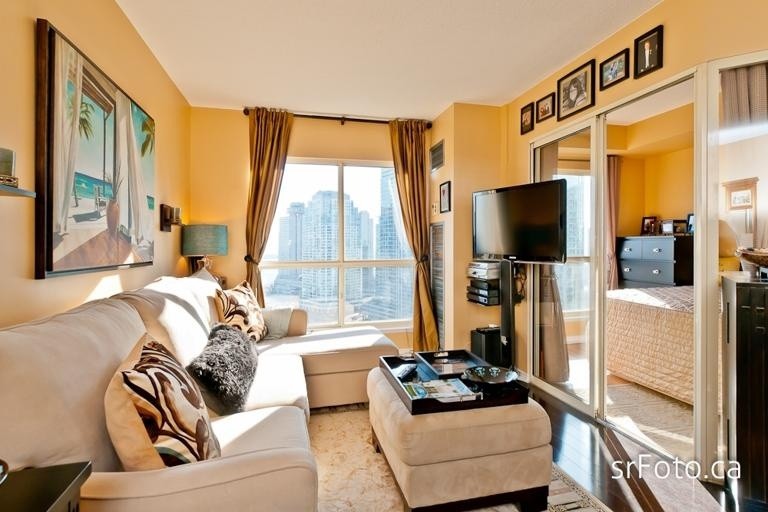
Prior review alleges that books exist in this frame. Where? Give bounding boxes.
[403,378,473,400]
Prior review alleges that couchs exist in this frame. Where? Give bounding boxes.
[0,269,400,512]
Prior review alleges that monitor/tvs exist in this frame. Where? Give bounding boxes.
[472,180,567,265]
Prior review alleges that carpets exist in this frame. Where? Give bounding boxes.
[605,381,695,469]
[309,403,616,512]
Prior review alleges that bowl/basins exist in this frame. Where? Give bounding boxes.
[736,248,768,266]
[462,364,519,386]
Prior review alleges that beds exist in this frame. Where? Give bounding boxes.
[604,285,696,409]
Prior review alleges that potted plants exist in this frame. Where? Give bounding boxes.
[104,161,125,235]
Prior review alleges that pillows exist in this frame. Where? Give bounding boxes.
[214,278,269,343]
[186,323,262,415]
[101,332,222,473]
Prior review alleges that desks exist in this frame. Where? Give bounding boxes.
[0,460,93,512]
[53,228,145,271]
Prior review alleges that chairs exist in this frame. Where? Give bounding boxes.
[93,185,109,218]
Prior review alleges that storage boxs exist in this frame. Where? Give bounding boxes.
[661,219,686,236]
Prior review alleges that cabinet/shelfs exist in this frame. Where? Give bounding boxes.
[721,268,768,511]
[615,232,695,289]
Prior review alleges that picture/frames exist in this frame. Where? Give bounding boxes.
[686,213,695,235]
[437,179,452,213]
[633,22,666,82]
[519,100,536,138]
[640,216,659,238]
[32,16,158,282]
[535,91,556,124]
[598,46,631,93]
[555,56,597,123]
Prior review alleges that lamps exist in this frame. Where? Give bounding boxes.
[181,223,230,279]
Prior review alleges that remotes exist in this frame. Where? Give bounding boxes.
[397,364,417,380]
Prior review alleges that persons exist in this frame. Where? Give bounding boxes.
[540,103,550,117]
[609,61,617,79]
[640,40,657,71]
[568,78,585,108]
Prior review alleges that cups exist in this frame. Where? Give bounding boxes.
[742,258,760,281]
[650,224,659,233]
